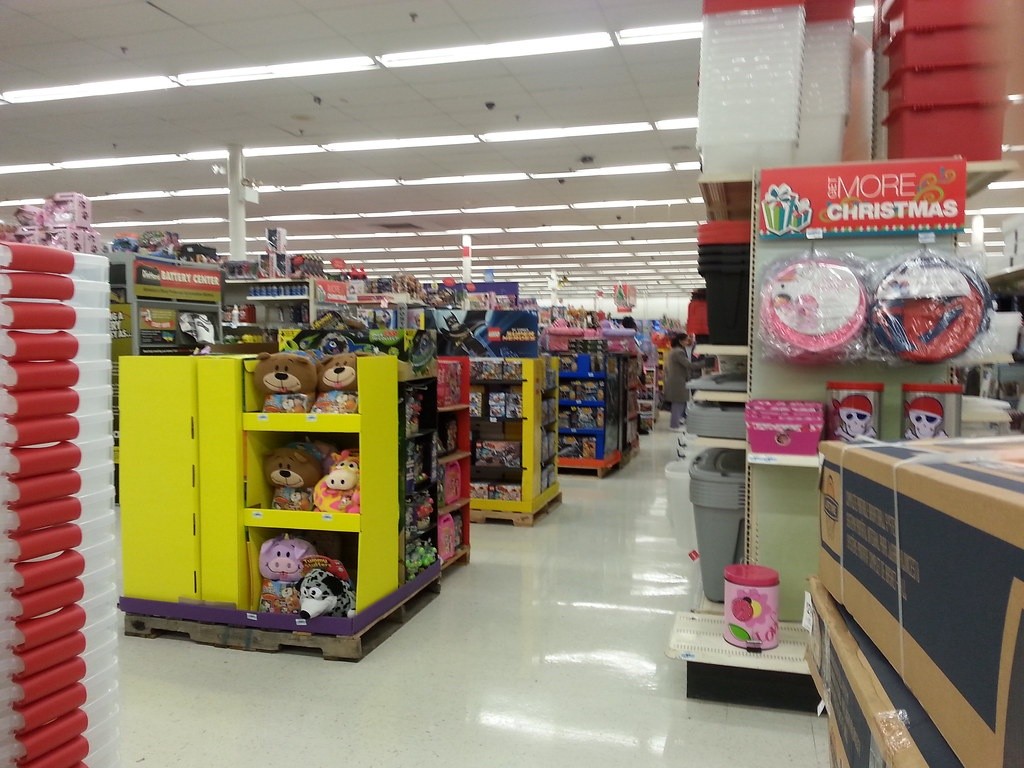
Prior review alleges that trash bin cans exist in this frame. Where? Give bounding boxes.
[688,447,746,604]
[686,219,751,345]
[721,564,779,653]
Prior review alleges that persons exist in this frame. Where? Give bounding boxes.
[663,333,715,432]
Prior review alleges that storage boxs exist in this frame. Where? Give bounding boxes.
[548,350,578,371]
[482,441,521,468]
[576,380,606,402]
[489,406,505,418]
[43,192,92,229]
[84,227,102,253]
[482,361,502,379]
[260,254,290,277]
[686,0,1024,768]
[559,436,597,460]
[469,391,484,418]
[570,406,605,429]
[45,229,85,253]
[488,392,506,405]
[470,483,489,499]
[506,394,524,419]
[489,483,521,500]
[470,361,483,380]
[503,361,522,379]
[265,227,287,254]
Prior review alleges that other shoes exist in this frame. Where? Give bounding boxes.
[669,428,685,432]
[680,417,685,425]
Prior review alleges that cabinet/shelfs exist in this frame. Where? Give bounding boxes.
[7,190,673,661]
[686,163,1017,713]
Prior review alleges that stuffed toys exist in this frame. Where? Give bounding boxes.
[252,349,369,413]
[257,533,356,619]
[262,438,360,514]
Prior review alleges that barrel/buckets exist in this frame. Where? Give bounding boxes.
[689,447,744,600]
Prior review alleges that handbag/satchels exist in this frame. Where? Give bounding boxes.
[684,388,695,415]
